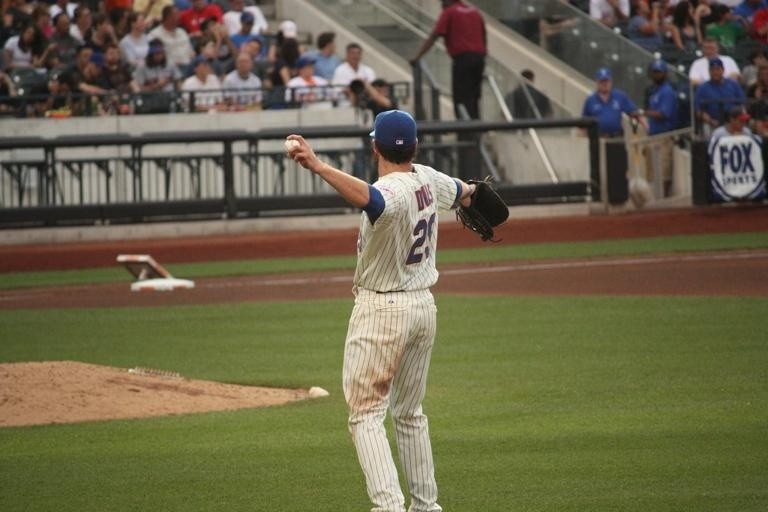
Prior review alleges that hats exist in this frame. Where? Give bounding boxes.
[296,57,316,69]
[596,69,611,82]
[709,58,722,66]
[278,20,297,39]
[370,110,417,145]
[649,60,667,73]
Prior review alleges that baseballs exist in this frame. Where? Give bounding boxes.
[283,139,300,154]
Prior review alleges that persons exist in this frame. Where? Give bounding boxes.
[347,75,399,214]
[538,0,766,209]
[407,1,491,124]
[0,0,399,121]
[284,108,512,512]
[497,68,556,121]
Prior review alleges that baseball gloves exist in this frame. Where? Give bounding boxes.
[453,175,510,242]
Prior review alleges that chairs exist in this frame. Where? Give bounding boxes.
[565,0,768,139]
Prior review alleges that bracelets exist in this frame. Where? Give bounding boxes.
[459,183,473,202]
